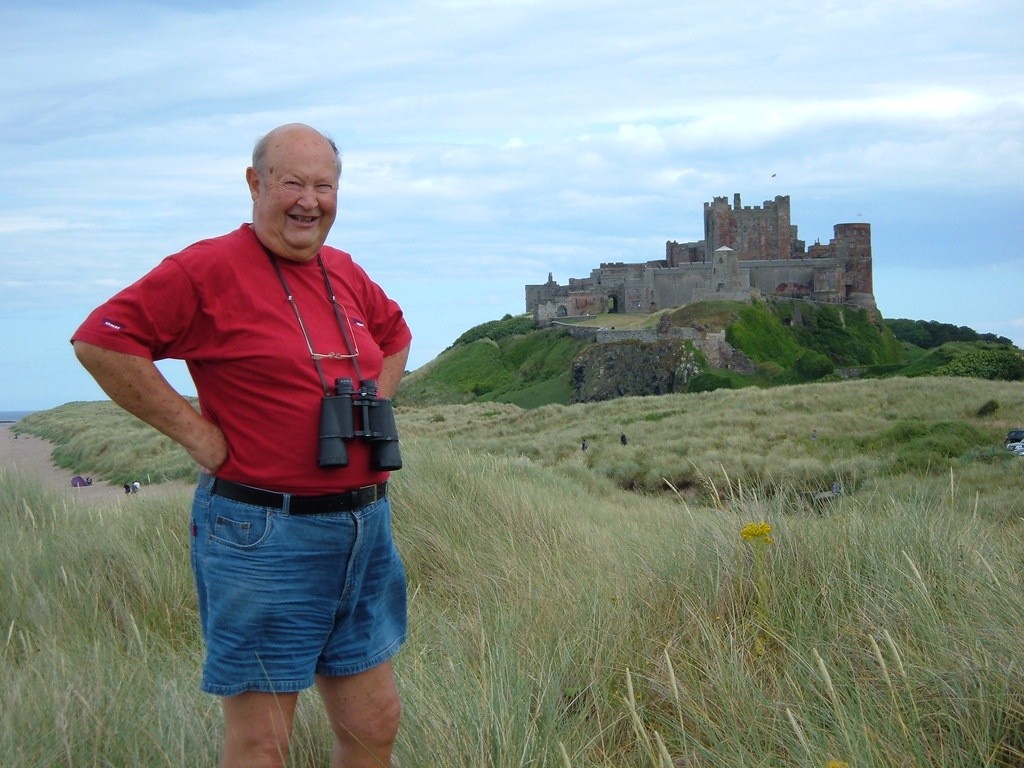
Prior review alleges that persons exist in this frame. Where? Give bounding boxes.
[124,481,141,493]
[71,123,412,768]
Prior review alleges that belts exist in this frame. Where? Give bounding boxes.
[199,471,388,516]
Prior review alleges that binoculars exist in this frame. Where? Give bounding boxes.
[318,377,402,471]
